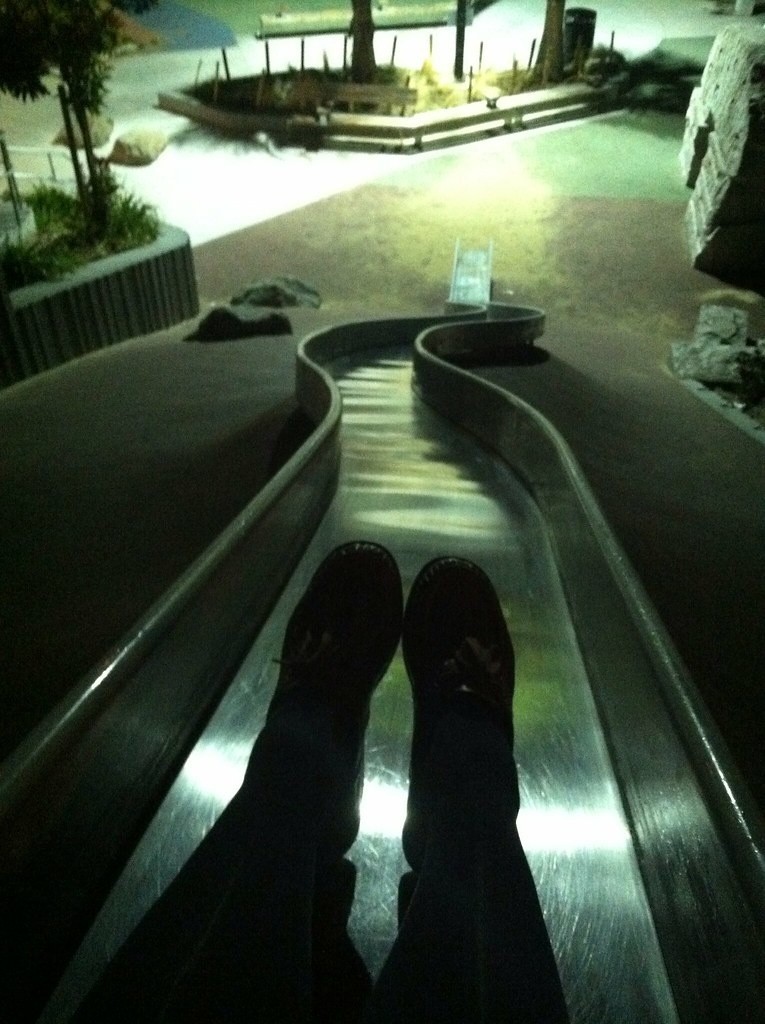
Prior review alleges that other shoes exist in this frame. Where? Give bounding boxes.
[246,540,401,805]
[401,556,520,818]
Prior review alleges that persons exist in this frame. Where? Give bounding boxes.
[68,541,569,1024]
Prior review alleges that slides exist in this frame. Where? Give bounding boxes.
[0,300,764,1024]
